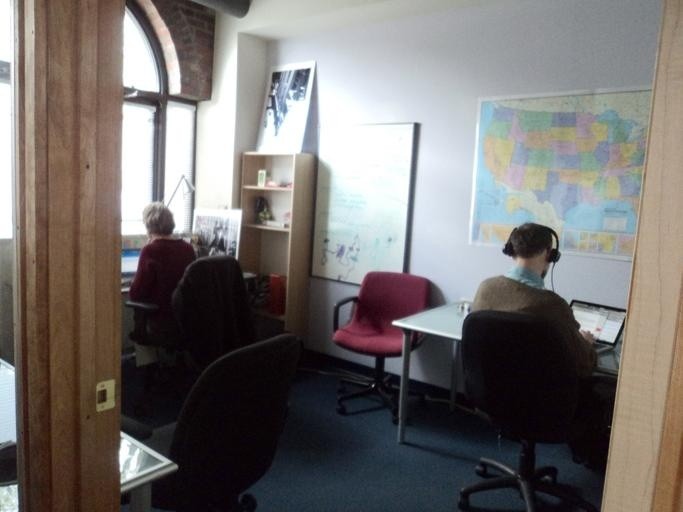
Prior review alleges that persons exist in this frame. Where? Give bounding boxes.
[130,201,197,341]
[467,222,597,379]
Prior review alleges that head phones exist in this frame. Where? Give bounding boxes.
[502,223,561,261]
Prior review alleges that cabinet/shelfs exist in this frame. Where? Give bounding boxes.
[237,151,316,333]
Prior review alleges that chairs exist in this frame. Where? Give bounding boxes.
[121,356,176,434]
[331,270,433,427]
[124,252,242,380]
[123,338,301,510]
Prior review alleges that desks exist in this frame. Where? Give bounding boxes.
[392,301,621,446]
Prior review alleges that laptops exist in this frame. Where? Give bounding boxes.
[121,249,141,286]
[569,300,626,354]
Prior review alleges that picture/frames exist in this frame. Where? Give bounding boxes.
[308,122,419,287]
[468,84,654,263]
[255,60,318,154]
